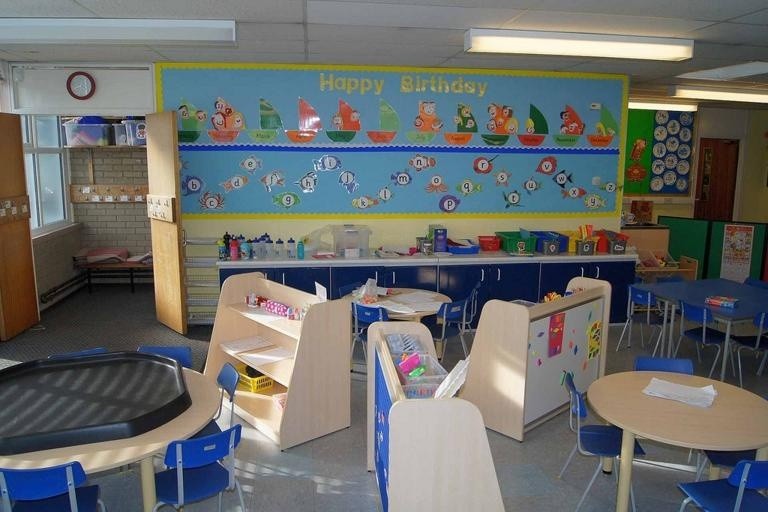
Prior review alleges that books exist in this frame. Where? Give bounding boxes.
[704,296,741,310]
[371,301,415,314]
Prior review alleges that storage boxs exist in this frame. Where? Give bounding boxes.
[121,118,146,147]
[63,121,111,149]
[111,123,128,147]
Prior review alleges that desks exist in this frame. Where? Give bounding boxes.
[1,352,225,510]
[586,364,767,511]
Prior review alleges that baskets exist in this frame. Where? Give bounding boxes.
[393,353,449,398]
[234,363,274,392]
[478,227,630,253]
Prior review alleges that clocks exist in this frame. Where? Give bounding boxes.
[65,70,95,101]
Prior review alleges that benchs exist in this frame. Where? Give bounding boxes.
[75,262,153,293]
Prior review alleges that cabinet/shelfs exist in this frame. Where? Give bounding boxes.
[540,261,637,325]
[438,263,541,306]
[332,267,438,300]
[361,316,505,511]
[218,264,331,302]
[457,273,614,445]
[199,270,357,451]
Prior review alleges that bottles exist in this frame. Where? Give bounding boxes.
[217,230,305,260]
[248,287,257,305]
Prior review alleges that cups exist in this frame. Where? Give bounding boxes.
[423,243,433,256]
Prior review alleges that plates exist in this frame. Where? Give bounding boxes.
[434,250,453,256]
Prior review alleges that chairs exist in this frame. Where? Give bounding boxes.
[615,273,767,390]
[337,274,485,378]
[138,343,194,369]
[145,423,251,511]
[673,459,767,511]
[47,345,109,357]
[155,360,242,471]
[560,368,648,511]
[1,456,108,511]
